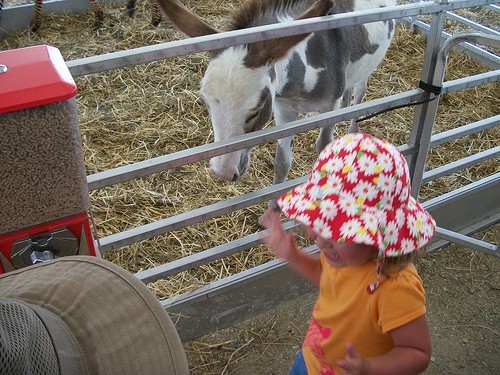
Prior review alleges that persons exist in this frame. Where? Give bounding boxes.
[259,133,437,374]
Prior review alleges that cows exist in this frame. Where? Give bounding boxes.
[159,0,396,228]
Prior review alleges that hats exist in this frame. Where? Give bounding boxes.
[0,255,189,375]
[276,132,436,294]
